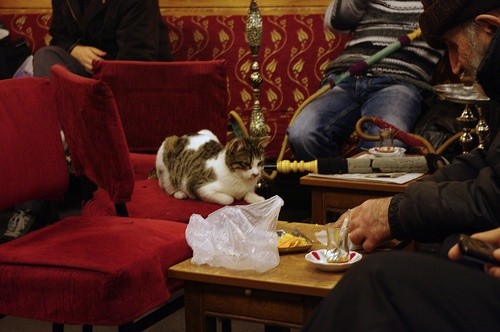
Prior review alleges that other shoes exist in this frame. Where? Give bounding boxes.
[0,200,60,245]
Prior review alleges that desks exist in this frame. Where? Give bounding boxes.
[300,149,428,224]
[167,221,411,332]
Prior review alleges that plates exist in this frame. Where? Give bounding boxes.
[369,146,407,157]
[278,230,314,254]
[305,249,362,272]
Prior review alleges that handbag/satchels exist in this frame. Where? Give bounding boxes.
[185,194,285,273]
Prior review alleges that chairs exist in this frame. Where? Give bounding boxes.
[0,58,251,332]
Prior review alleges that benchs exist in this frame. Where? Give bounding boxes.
[0,13,458,159]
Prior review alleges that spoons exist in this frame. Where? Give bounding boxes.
[327,208,351,262]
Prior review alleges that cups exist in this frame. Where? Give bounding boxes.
[325,223,350,263]
[377,129,394,152]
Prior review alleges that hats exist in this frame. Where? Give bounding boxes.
[419,0,500,50]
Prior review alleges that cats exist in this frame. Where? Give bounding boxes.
[155,128,273,205]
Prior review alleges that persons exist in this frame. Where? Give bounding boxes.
[32,0,172,80]
[286,0,446,161]
[334,0,500,332]
